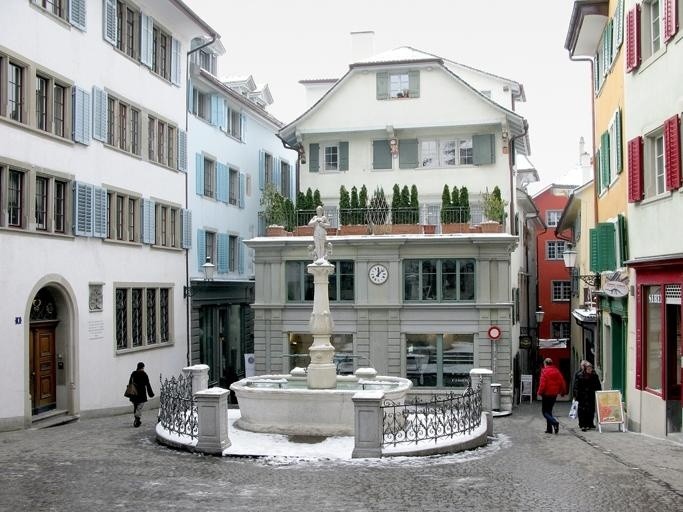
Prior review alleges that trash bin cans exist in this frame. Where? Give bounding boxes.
[490,384,501,411]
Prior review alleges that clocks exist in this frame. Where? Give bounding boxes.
[368,263,389,285]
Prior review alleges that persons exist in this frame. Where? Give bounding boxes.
[308,205,330,264]
[127,362,155,427]
[537,358,567,434]
[573,362,602,430]
[572,359,596,428]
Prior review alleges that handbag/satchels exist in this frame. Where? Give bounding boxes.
[569,401,579,420]
[124,383,139,397]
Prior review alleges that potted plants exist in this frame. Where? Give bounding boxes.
[439,184,471,233]
[480,185,508,233]
[255,182,323,236]
[338,184,422,235]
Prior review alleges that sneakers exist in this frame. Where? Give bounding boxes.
[545,422,558,433]
[581,424,596,430]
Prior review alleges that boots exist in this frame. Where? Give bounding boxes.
[134,417,141,427]
